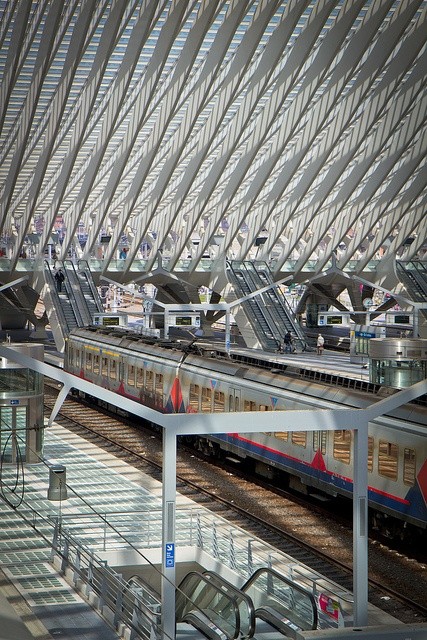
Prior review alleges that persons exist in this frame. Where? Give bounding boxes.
[283,329,295,354]
[316,332,324,354]
[54,269,65,294]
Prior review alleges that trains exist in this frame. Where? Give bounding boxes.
[63,324,426,564]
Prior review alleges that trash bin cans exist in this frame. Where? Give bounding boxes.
[48,464,66,501]
